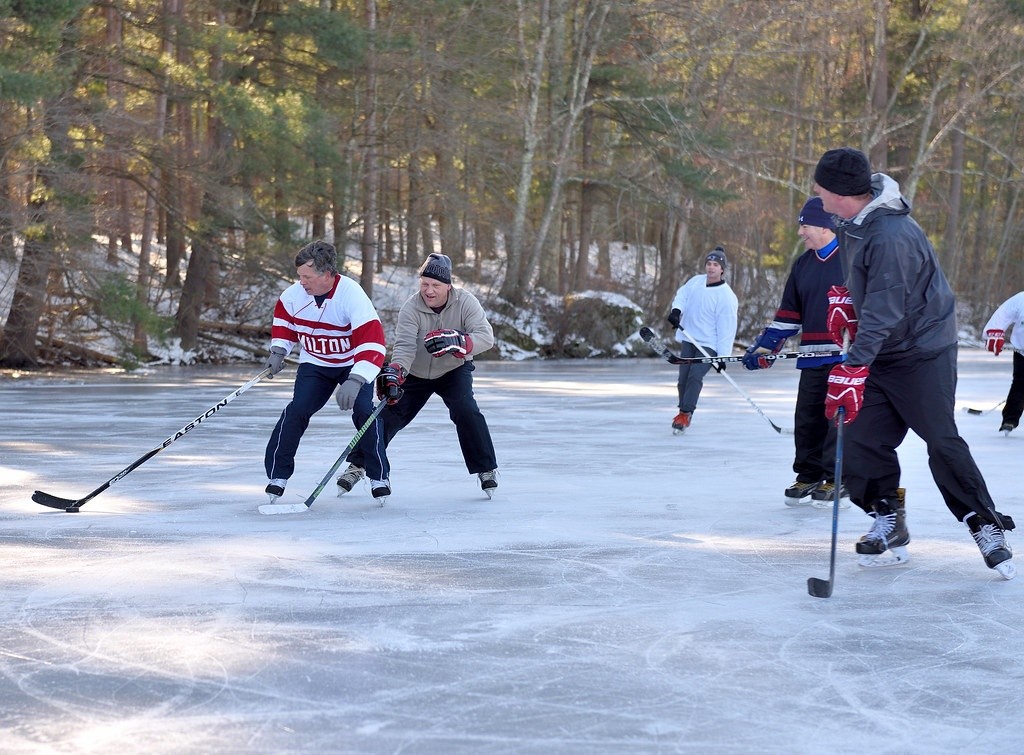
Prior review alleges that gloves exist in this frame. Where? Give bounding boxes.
[424,329,467,358]
[826,284,857,346]
[668,308,681,328]
[824,364,868,426]
[741,330,787,370]
[336,374,365,411]
[713,361,726,373]
[984,329,1006,355]
[263,345,287,379]
[377,367,404,405]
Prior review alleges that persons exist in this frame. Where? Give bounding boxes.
[336,253,500,500]
[743,195,862,509]
[265,240,392,508]
[981,290,1024,437]
[667,246,738,436]
[812,148,1016,580]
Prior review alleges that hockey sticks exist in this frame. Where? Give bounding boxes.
[677,324,794,436]
[806,329,851,600]
[962,399,1008,416]
[257,395,388,516]
[638,326,843,366]
[31,361,289,511]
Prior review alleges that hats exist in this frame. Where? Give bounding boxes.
[814,147,871,196]
[705,246,727,272]
[420,253,453,284]
[798,197,835,229]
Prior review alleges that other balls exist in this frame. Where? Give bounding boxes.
[65,507,79,512]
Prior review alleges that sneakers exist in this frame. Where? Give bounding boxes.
[266,478,288,503]
[963,511,1018,580]
[337,463,366,497]
[671,411,692,435]
[999,423,1014,437]
[370,478,390,506]
[478,469,499,499]
[784,481,823,506]
[811,482,852,509]
[856,488,911,568]
